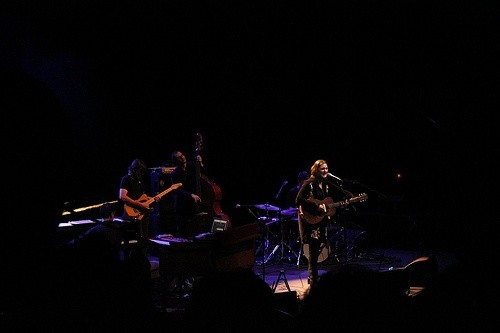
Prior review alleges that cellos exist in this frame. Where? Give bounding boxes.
[176,133,232,240]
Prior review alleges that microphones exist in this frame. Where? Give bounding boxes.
[276,181,288,199]
[236,204,255,207]
[328,172,342,184]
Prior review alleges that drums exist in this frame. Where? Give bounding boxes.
[303,241,331,263]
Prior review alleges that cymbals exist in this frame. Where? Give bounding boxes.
[276,206,298,216]
[255,204,281,210]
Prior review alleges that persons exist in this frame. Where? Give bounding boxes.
[181,237,276,333]
[118,158,161,233]
[170,151,206,230]
[295,160,349,285]
[287,170,310,250]
[84,203,124,245]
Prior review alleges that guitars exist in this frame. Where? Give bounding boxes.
[124,183,182,221]
[299,192,368,225]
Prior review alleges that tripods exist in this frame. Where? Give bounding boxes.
[271,197,298,301]
